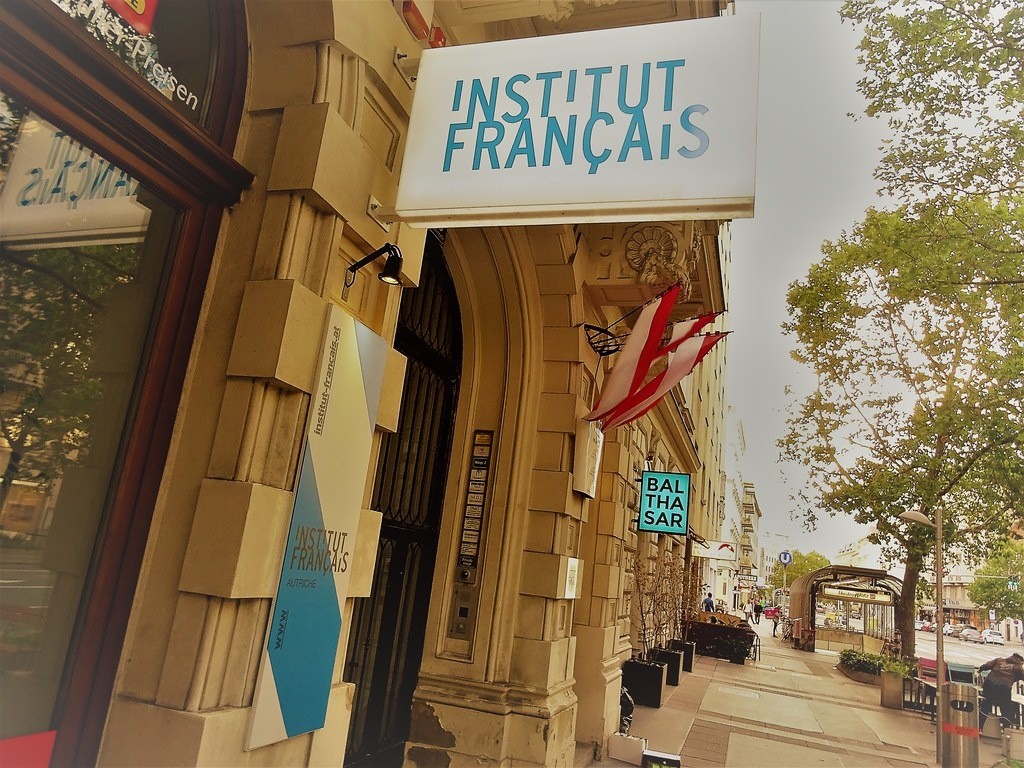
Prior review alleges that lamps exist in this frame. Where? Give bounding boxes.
[341,242,403,302]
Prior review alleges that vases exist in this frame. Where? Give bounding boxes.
[622,639,711,709]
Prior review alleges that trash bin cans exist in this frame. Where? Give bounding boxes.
[936,682,982,768]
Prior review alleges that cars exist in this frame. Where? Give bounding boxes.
[981,629,1004,646]
[851,612,861,619]
[816,604,825,613]
[958,629,983,644]
[915,619,978,637]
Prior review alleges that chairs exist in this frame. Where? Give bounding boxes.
[911,657,992,716]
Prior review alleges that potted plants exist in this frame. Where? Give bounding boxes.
[880,660,909,710]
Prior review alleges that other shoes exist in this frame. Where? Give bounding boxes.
[979,729,983,735]
[773,635,777,637]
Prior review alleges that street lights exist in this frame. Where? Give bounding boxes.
[897,506,946,765]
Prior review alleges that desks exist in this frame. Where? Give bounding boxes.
[913,677,1024,730]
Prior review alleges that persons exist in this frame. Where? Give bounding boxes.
[773,604,782,637]
[977,653,1024,738]
[742,600,755,624]
[702,593,714,612]
[754,602,762,625]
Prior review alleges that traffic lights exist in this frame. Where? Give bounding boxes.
[1007,581,1012,590]
[1013,581,1017,590]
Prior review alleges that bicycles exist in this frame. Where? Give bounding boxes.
[776,618,794,642]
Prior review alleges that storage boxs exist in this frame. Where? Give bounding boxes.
[607,731,683,768]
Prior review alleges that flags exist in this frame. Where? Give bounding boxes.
[581,286,728,434]
